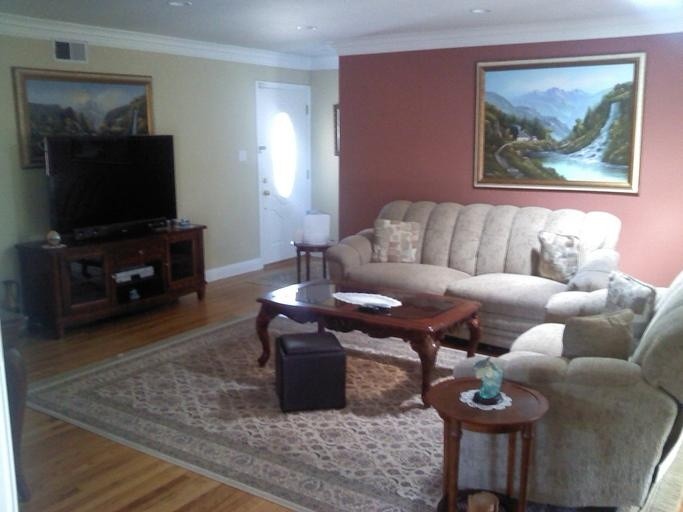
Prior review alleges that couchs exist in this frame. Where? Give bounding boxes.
[447,271,682,512]
[324,197,622,351]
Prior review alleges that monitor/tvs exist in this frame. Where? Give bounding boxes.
[44,134,177,245]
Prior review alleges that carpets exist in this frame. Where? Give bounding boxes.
[26,312,507,512]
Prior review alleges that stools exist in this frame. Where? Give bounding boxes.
[273,332,346,414]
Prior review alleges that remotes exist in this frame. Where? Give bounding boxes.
[358,302,392,313]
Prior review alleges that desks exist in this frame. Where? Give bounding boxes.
[419,375,551,512]
[289,236,331,284]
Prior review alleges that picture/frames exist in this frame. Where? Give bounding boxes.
[471,50,649,197]
[330,102,343,157]
[8,61,157,173]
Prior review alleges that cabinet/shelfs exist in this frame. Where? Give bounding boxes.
[12,217,209,339]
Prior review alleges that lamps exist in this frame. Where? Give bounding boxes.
[292,0,317,46]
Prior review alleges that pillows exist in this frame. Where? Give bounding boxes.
[598,270,656,340]
[368,215,424,267]
[560,307,634,362]
[534,228,582,284]
